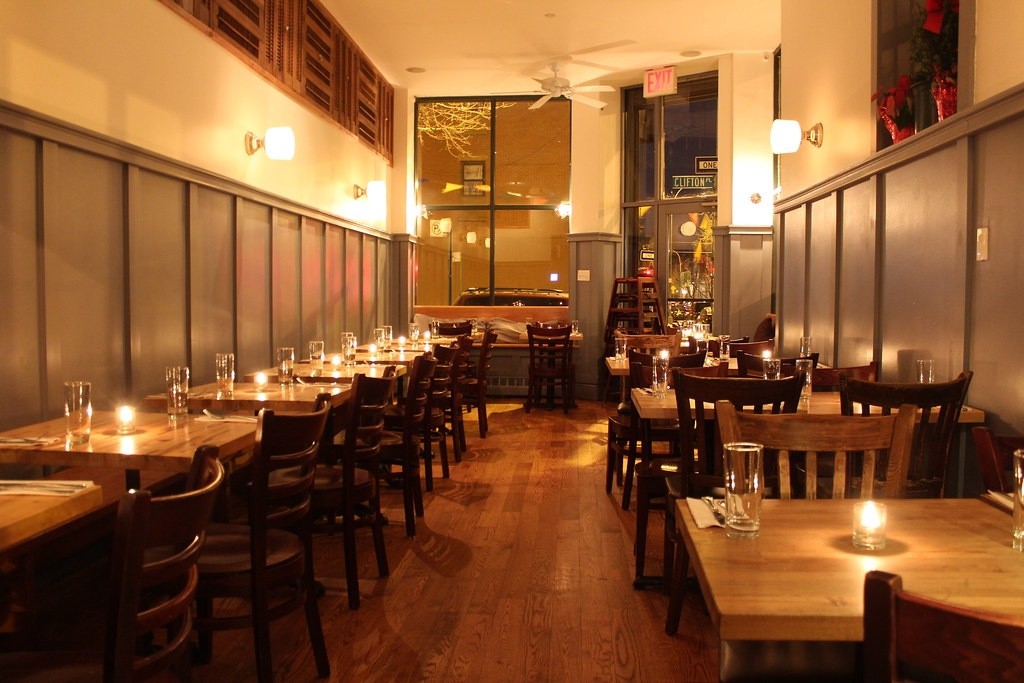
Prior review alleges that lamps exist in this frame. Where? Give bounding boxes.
[354,180,386,201]
[244,128,296,159]
[769,119,823,155]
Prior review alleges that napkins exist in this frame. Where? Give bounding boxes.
[686,496,725,528]
[0,480,93,497]
[194,415,258,423]
[0,437,61,447]
[636,388,652,395]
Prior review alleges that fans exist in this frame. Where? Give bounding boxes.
[489,66,615,111]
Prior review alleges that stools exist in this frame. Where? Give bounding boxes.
[603,275,667,355]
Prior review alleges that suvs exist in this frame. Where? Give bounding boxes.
[439,287,569,306]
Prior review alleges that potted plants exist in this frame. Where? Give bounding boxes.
[869,0,957,143]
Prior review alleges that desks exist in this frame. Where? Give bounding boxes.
[0,411,256,656]
[245,363,407,383]
[606,357,829,487]
[299,339,456,398]
[630,388,983,588]
[674,500,1024,683]
[0,479,103,554]
[470,333,583,396]
[145,382,352,498]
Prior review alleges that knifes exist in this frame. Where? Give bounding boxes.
[638,386,651,396]
[0,483,85,488]
[702,496,725,526]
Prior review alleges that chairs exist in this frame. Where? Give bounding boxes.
[269,366,389,610]
[0,445,223,683]
[334,324,578,610]
[145,394,331,683]
[604,330,1024,683]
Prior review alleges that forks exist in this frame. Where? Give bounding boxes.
[202,377,336,423]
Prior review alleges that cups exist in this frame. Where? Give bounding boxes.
[693,323,731,362]
[652,355,669,398]
[851,500,888,551]
[614,338,628,362]
[63,381,92,445]
[722,442,764,540]
[525,318,533,334]
[166,366,189,415]
[1012,449,1024,553]
[762,359,781,380]
[796,359,813,399]
[115,406,137,434]
[916,359,935,384]
[571,321,579,335]
[799,337,812,357]
[216,318,478,400]
